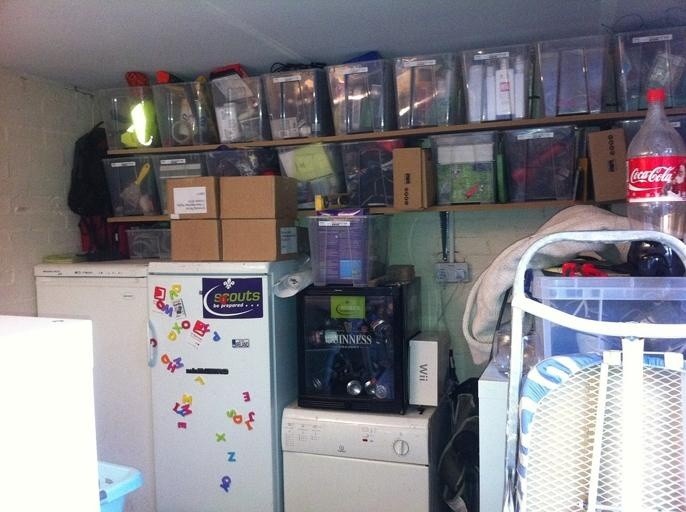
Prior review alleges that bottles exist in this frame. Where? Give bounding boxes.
[622,84,686,276]
[485,55,526,122]
[307,302,395,399]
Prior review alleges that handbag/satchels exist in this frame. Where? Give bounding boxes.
[68,127,113,216]
[79,218,129,260]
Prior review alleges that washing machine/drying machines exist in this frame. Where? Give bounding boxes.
[279,398,440,512]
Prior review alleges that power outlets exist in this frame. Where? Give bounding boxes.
[434,263,468,284]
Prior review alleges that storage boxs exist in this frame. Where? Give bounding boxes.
[529,266,686,363]
[303,214,392,287]
[93,29,685,264]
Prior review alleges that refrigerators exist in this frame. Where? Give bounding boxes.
[146,256,301,512]
[300,272,424,417]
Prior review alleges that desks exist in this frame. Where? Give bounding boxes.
[477,351,508,511]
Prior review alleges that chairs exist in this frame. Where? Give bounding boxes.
[517,352,684,510]
